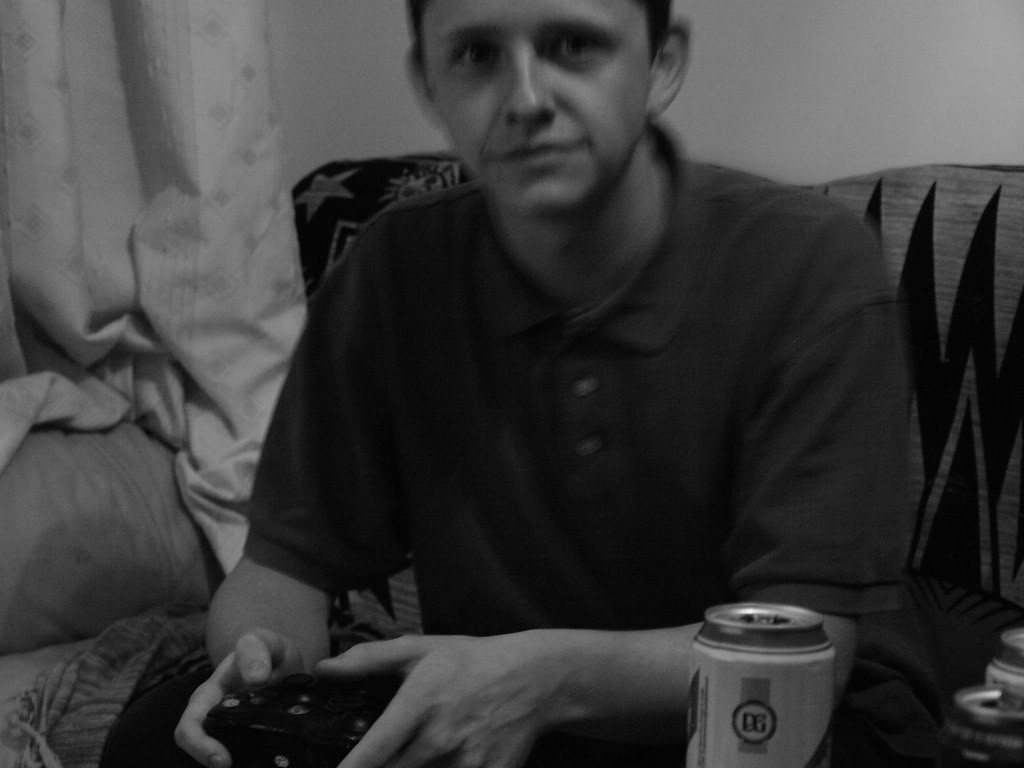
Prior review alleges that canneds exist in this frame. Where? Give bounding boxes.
[681,601,835,768]
[936,625,1024,768]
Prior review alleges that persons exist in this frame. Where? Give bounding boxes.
[100,0,968,768]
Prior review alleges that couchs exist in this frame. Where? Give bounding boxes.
[0,151,1024,768]
